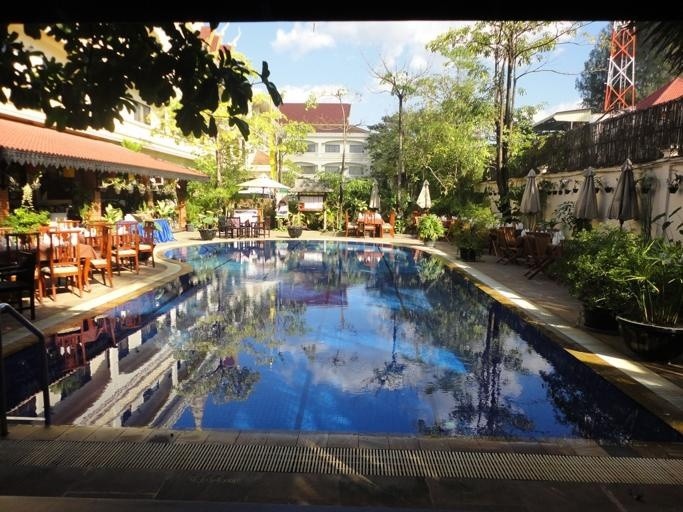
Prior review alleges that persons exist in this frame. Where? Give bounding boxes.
[64,194,83,226]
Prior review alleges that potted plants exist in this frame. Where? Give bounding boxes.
[284,212,305,238]
[568,218,683,360]
[184,178,224,239]
[636,172,683,194]
[544,176,612,195]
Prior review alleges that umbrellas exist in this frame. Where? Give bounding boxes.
[572,165,599,229]
[519,168,540,232]
[233,173,289,220]
[368,180,381,227]
[607,158,640,232]
[414,178,432,215]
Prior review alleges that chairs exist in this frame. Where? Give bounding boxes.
[0,220,155,320]
[219,216,271,239]
[55,300,146,372]
[345,210,395,239]
[412,211,565,281]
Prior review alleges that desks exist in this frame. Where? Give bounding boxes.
[137,219,175,242]
